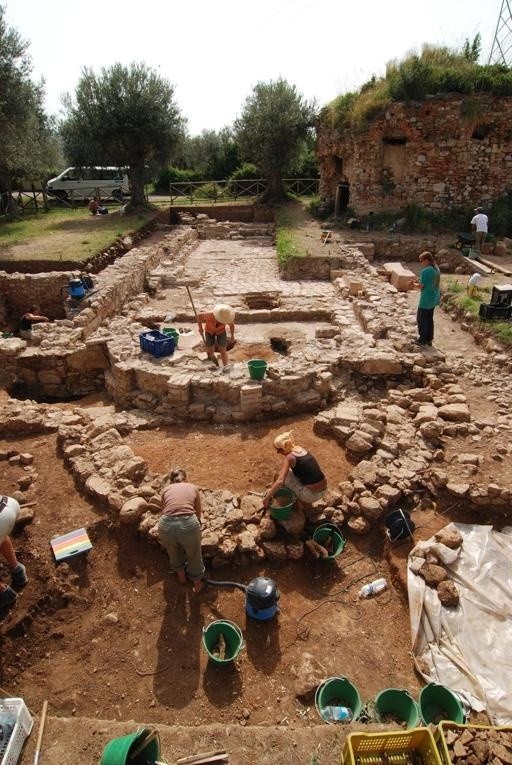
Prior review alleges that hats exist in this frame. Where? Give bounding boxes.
[212,302,236,325]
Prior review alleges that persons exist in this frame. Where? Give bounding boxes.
[263,431,329,513]
[157,468,211,595]
[469,206,489,252]
[0,494,30,605]
[18,303,51,340]
[412,250,441,348]
[89,196,100,215]
[196,303,236,373]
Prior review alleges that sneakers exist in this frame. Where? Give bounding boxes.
[9,563,29,589]
[410,337,433,347]
[0,584,20,610]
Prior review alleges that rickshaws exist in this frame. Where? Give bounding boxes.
[456,231,475,249]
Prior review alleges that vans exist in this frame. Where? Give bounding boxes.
[46,166,133,200]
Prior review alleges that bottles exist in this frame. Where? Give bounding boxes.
[358,577,388,598]
[319,705,353,723]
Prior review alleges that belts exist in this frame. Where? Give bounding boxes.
[0,495,9,513]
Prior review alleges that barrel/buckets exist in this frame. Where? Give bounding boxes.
[100,725,160,765]
[201,620,245,665]
[313,523,347,574]
[263,486,296,519]
[248,359,267,379]
[165,332,179,346]
[375,687,421,729]
[314,674,362,726]
[420,682,465,727]
[163,327,176,334]
